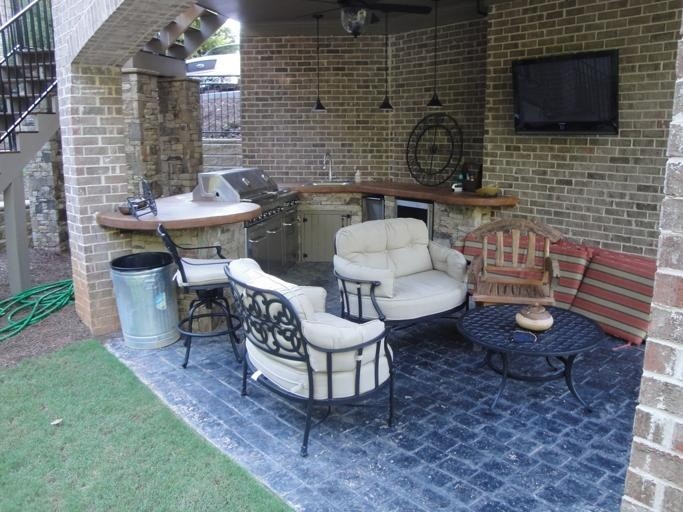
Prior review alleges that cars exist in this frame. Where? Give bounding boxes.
[179,52,240,94]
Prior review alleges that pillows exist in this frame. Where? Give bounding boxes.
[453,227,527,304]
[569,248,658,350]
[523,236,594,310]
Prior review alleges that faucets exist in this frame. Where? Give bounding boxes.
[323,151,333,181]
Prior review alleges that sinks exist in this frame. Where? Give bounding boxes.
[303,181,349,187]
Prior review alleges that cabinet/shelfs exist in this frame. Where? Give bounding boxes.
[301,210,352,262]
[244,208,299,277]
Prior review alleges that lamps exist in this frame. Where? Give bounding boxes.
[379,10,393,111]
[311,14,326,112]
[341,7,366,37]
[426,0,443,107]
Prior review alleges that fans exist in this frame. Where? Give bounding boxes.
[295,0,431,16]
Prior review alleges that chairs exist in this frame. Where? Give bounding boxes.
[470,218,562,351]
[332,217,473,336]
[157,223,242,369]
[223,258,394,457]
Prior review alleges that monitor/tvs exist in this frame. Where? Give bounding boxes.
[511,48,621,138]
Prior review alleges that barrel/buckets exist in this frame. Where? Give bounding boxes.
[109,252,180,348]
